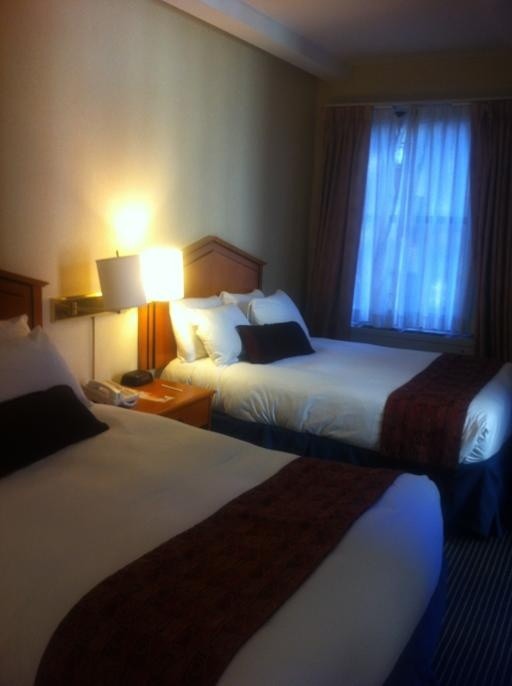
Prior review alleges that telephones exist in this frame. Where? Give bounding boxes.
[85,379,139,406]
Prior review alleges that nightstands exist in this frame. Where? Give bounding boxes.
[118,377,217,432]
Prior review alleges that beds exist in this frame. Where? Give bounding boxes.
[137,235,512,540]
[0,268,446,685]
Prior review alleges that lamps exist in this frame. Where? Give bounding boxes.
[49,250,146,322]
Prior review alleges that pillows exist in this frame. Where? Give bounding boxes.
[0,315,109,481]
[171,290,316,366]
